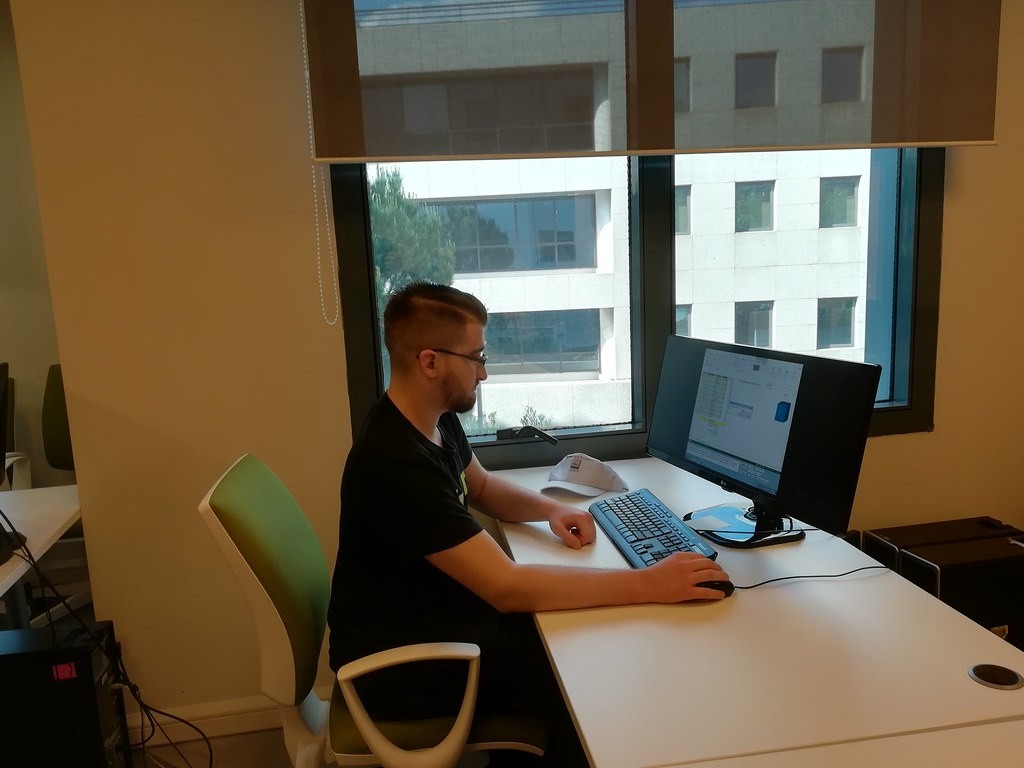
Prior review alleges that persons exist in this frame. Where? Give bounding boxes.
[327,281,729,768]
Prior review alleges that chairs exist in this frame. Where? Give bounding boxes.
[0,358,33,494]
[196,452,549,768]
[26,363,93,629]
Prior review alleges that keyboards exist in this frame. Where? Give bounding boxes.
[588,488,718,570]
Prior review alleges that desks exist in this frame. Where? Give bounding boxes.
[0,484,81,598]
[493,457,1024,767]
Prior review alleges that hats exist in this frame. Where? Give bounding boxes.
[539,451,630,498]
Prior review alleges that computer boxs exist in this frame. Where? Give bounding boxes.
[862,515,1024,653]
[0,620,134,768]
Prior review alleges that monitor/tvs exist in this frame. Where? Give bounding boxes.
[646,334,883,550]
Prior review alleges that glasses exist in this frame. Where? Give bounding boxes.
[416,347,487,368]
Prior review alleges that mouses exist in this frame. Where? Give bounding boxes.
[694,580,735,597]
